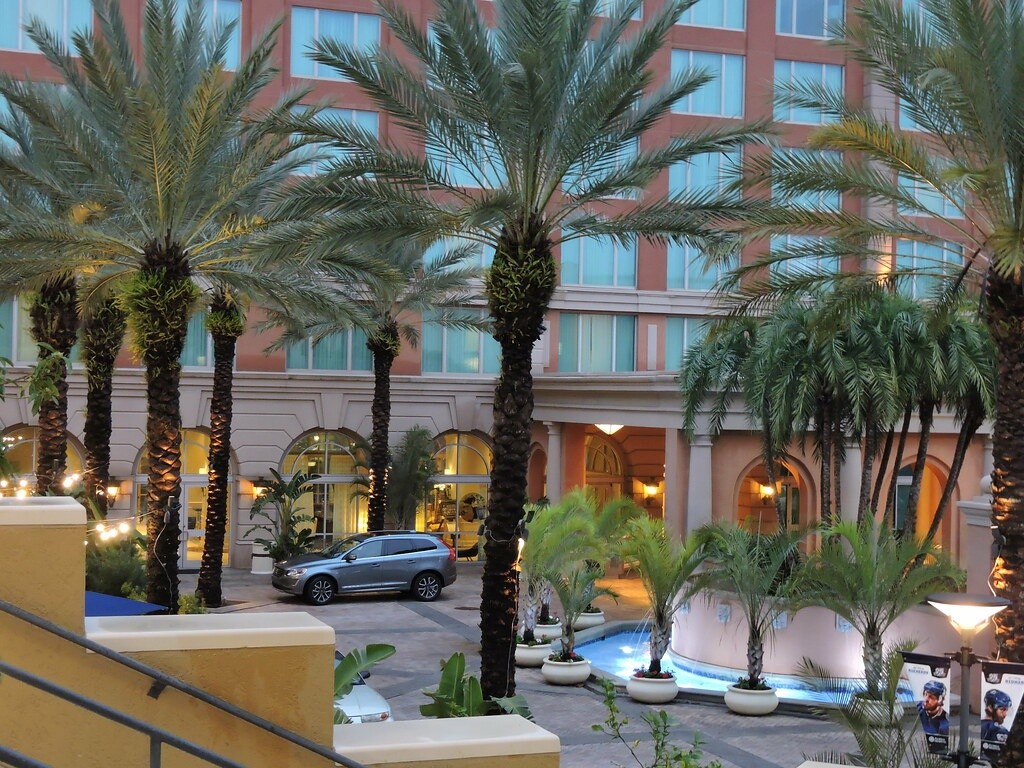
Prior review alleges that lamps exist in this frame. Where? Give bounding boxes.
[253,475,272,500]
[760,478,774,505]
[644,476,660,505]
[107,476,124,507]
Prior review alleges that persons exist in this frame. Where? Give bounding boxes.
[917,680,948,752]
[981,688,1012,761]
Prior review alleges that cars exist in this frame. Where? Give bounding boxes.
[271,529,457,605]
[332,648,394,725]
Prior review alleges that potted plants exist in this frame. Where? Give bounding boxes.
[613,512,732,705]
[789,506,969,727]
[702,512,834,715]
[514,481,650,686]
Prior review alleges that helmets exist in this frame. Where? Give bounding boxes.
[984,689,1012,710]
[923,680,947,706]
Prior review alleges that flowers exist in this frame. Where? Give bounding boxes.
[548,649,582,663]
[633,667,675,680]
[546,611,560,626]
[739,678,768,689]
[515,634,556,647]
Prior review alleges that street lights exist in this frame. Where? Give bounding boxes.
[898,589,1023,768]
